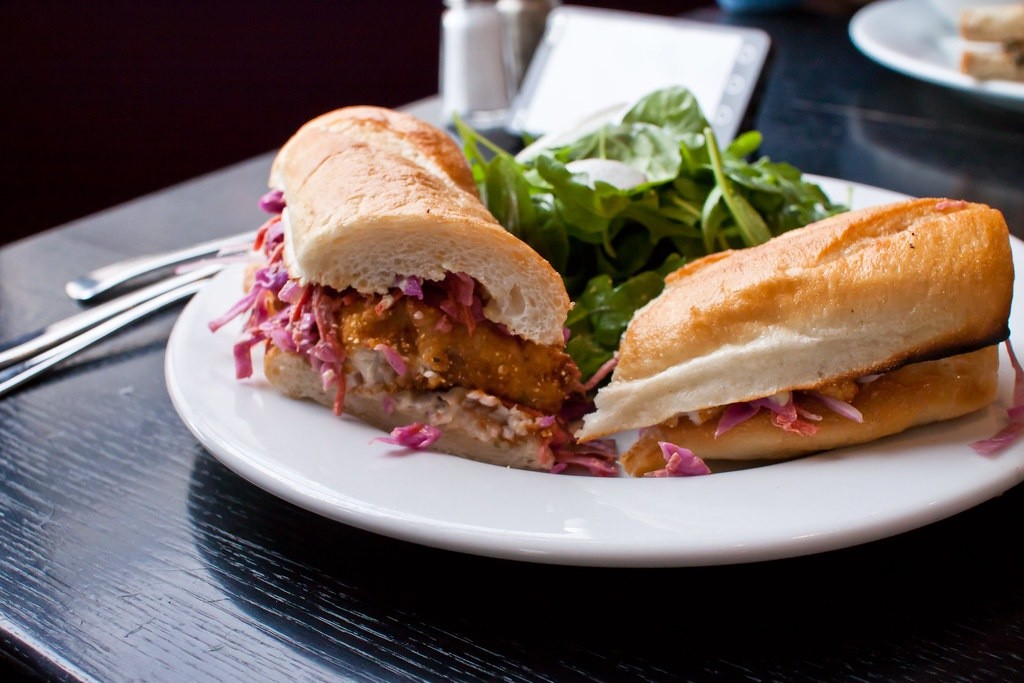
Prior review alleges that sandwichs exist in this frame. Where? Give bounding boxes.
[210,106,584,472]
[956,5,1024,84]
[573,195,1014,476]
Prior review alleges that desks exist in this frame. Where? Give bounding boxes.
[0,0,1024,683]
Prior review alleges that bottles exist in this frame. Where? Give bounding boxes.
[438,0,507,121]
[496,0,551,103]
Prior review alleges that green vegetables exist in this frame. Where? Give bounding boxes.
[450,85,854,393]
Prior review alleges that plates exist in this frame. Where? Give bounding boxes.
[848,0,1024,113]
[163,159,1024,568]
